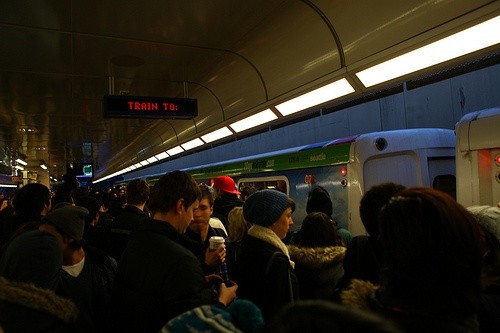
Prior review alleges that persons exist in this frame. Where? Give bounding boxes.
[0,169,500,333]
[232,189,296,322]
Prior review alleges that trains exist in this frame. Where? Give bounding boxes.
[107,104,500,254]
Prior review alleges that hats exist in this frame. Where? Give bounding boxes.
[160,298,264,333]
[42,202,90,240]
[214,175,241,194]
[0,231,63,295]
[306,186,333,216]
[242,189,289,227]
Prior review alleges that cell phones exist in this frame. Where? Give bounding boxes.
[208,278,233,292]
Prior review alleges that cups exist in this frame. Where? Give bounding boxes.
[209,236,225,252]
